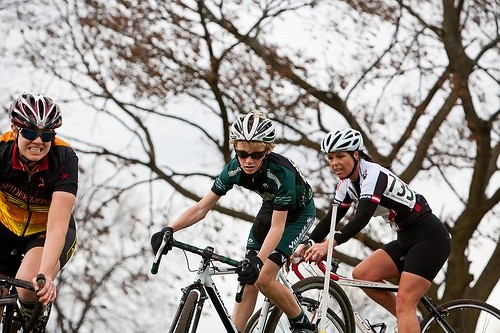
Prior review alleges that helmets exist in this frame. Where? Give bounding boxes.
[9,93,62,131]
[230,114,275,146]
[321,128,361,152]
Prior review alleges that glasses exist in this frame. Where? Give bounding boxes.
[234,144,267,159]
[16,126,56,142]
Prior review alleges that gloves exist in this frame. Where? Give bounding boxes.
[151,226,172,256]
[236,256,264,286]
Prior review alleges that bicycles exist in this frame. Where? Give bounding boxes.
[0,247,49,333]
[150,230,355,333]
[244,244,500,333]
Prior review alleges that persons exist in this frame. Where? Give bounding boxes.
[285,129,452,333]
[0,93,78,332]
[151,110,318,333]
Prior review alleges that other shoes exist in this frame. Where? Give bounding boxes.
[291,324,318,333]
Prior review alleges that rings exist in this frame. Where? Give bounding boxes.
[51,291,54,293]
[312,255,317,258]
